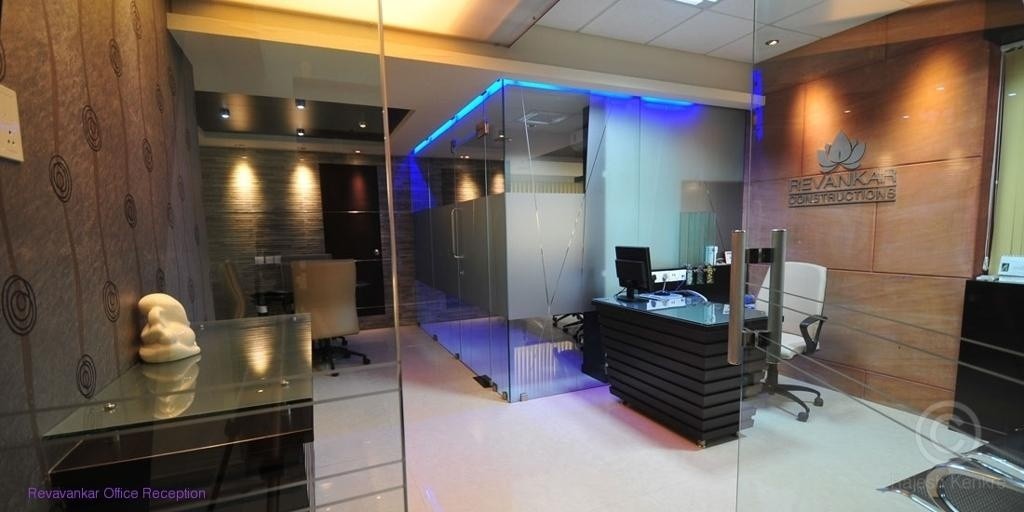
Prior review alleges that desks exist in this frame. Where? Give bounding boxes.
[592,292,784,449]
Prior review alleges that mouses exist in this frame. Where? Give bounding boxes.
[655,290,669,295]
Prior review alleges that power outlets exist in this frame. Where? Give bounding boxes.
[0,83,24,165]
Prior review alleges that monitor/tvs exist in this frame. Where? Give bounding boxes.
[615,246,651,302]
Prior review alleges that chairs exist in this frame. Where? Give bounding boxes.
[749,260,827,422]
[221,258,370,378]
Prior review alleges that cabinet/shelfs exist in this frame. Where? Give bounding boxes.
[40,313,316,512]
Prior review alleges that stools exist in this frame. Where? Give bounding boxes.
[874,435,1024,511]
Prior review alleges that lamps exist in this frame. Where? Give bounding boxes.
[295,98,307,110]
[219,107,230,119]
